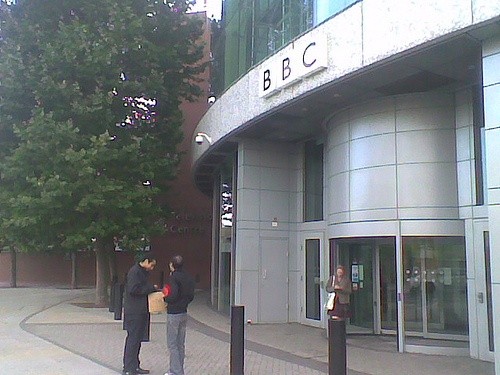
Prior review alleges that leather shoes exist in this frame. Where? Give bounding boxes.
[122,372,138,375]
[136,368,150,374]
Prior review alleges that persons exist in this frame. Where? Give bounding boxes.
[122,253,160,375]
[162,255,195,375]
[326,266,352,320]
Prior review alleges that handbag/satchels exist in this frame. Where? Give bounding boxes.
[324,293,335,310]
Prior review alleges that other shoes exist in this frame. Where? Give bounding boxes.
[164,373,176,375]
